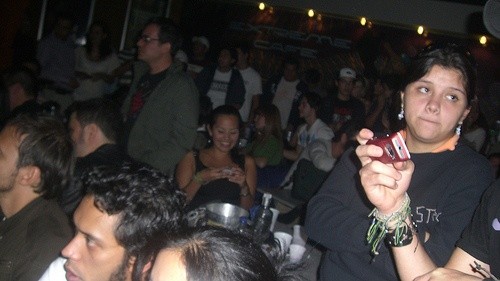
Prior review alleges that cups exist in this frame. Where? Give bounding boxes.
[274,231,292,254]
[220,163,237,177]
[288,243,306,263]
[269,208,279,231]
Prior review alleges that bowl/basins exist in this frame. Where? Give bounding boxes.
[205,203,248,228]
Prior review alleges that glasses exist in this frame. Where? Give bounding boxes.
[141,36,160,43]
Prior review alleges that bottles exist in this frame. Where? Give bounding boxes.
[292,225,309,246]
[238,217,248,236]
[252,193,274,246]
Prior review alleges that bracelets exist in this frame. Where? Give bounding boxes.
[363,193,419,263]
[240,191,252,198]
[192,171,207,187]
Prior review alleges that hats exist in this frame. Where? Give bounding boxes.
[340,68,356,78]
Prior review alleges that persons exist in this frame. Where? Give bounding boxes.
[129,219,282,281]
[1,15,405,229]
[1,100,75,281]
[39,159,210,279]
[353,125,500,281]
[305,40,498,281]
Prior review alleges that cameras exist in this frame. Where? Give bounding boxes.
[367,132,411,163]
